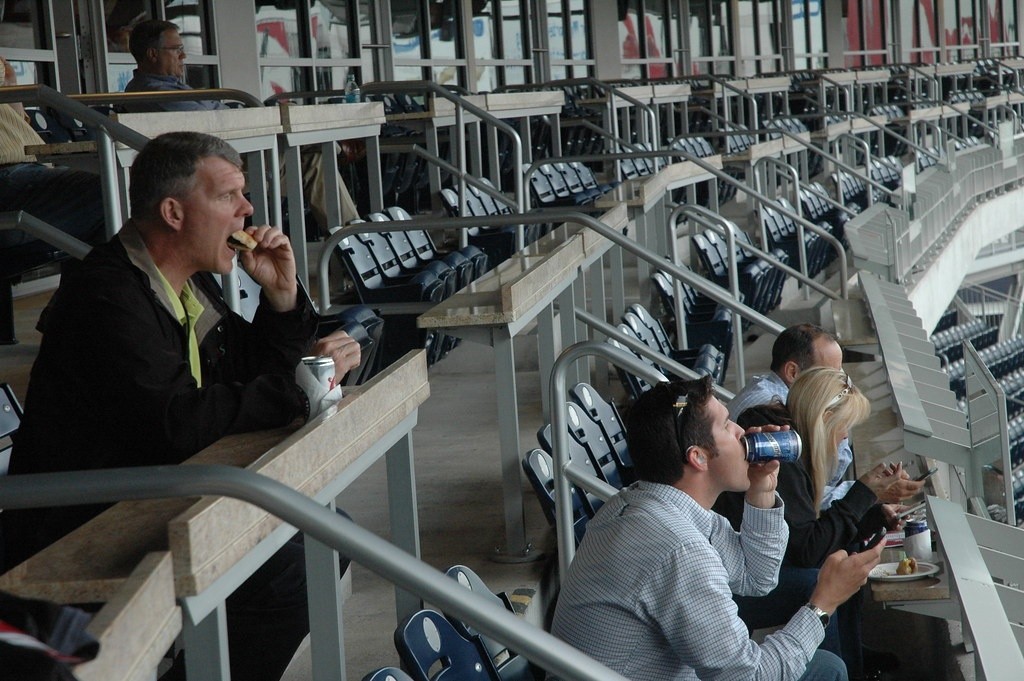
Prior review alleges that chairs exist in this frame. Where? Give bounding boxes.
[2,55,1024,681]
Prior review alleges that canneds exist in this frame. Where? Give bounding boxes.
[300,355,336,420]
[739,430,802,464]
[903,519,930,563]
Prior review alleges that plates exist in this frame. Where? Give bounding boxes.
[868,561,940,582]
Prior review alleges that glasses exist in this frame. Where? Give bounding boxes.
[157,45,184,54]
[656,382,692,465]
[825,372,852,409]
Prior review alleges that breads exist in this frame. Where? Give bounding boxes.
[895,557,918,575]
[227,229,258,251]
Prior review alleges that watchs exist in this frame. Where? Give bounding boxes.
[805,603,830,629]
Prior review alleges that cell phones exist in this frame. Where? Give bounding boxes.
[895,500,927,519]
[914,467,938,482]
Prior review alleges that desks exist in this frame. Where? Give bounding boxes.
[0,70,1024,681]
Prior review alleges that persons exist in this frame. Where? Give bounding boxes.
[123,20,361,232]
[725,325,917,531]
[0,132,361,680]
[544,372,887,681]
[0,0,107,275]
[710,366,926,681]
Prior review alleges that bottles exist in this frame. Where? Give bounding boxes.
[346,75,360,104]
